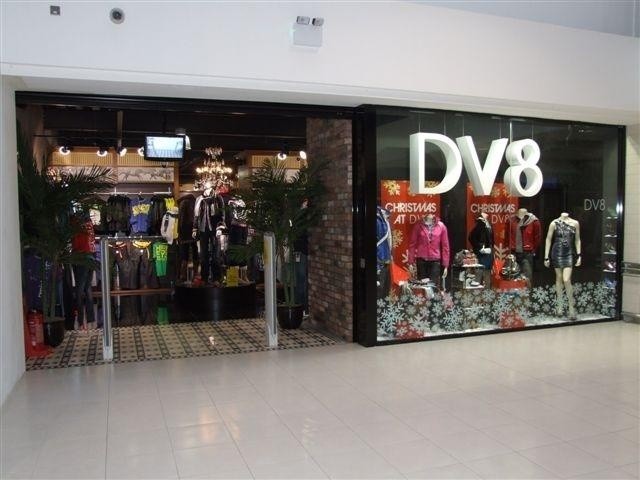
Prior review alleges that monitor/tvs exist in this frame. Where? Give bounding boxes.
[144,134,186,161]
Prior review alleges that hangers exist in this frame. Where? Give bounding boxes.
[112,191,172,202]
[110,235,164,243]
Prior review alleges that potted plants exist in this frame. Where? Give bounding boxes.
[18,141,118,348]
[215,158,331,328]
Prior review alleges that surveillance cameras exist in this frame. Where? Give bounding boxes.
[109,7,125,24]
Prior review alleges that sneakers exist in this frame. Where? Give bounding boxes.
[605,223,616,287]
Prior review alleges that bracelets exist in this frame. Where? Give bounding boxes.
[544,258,549,261]
[576,252,584,256]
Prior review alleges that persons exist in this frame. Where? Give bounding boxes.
[408,213,450,293]
[467,212,495,289]
[191,180,226,287]
[227,194,252,283]
[504,207,543,290]
[376,208,393,337]
[70,209,97,332]
[543,212,584,321]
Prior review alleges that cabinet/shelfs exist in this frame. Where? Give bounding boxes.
[603,216,617,290]
[451,264,487,308]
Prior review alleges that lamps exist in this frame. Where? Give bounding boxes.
[32,133,191,156]
[277,150,287,160]
